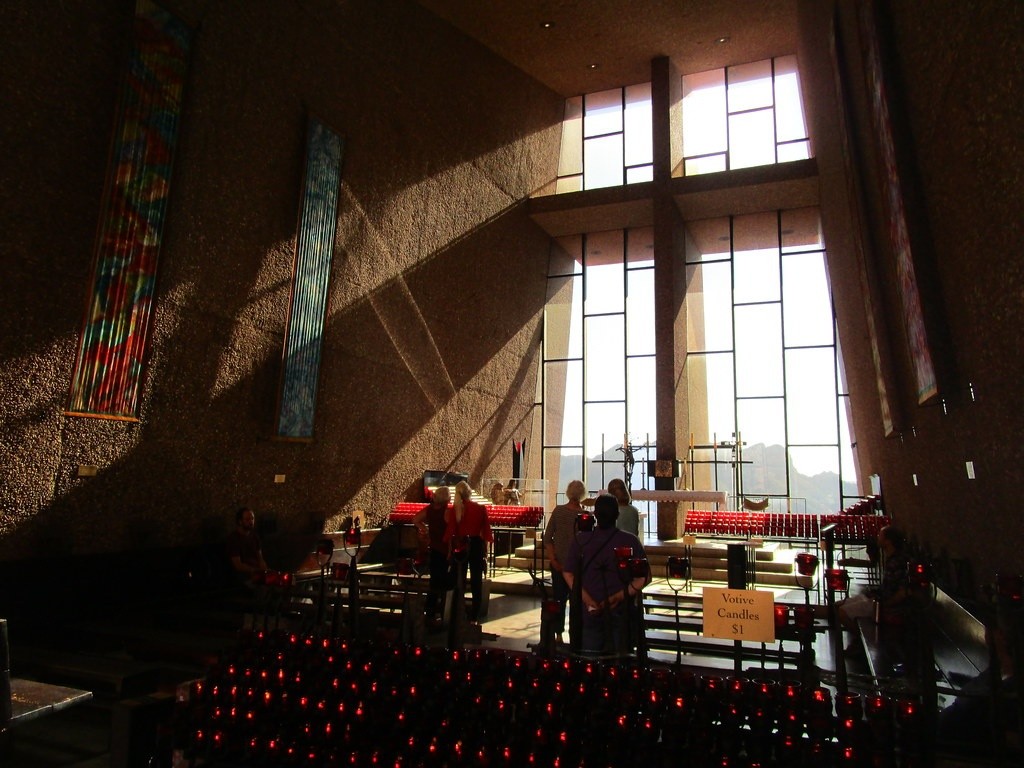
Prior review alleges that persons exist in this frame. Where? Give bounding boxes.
[221,507,267,634]
[562,494,652,652]
[608,479,639,536]
[412,480,493,636]
[545,481,587,643]
[836,526,911,658]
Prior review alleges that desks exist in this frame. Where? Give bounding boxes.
[0,675,94,734]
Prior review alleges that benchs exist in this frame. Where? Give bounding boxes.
[254,603,382,639]
[360,569,453,627]
[406,573,489,620]
[309,578,435,631]
